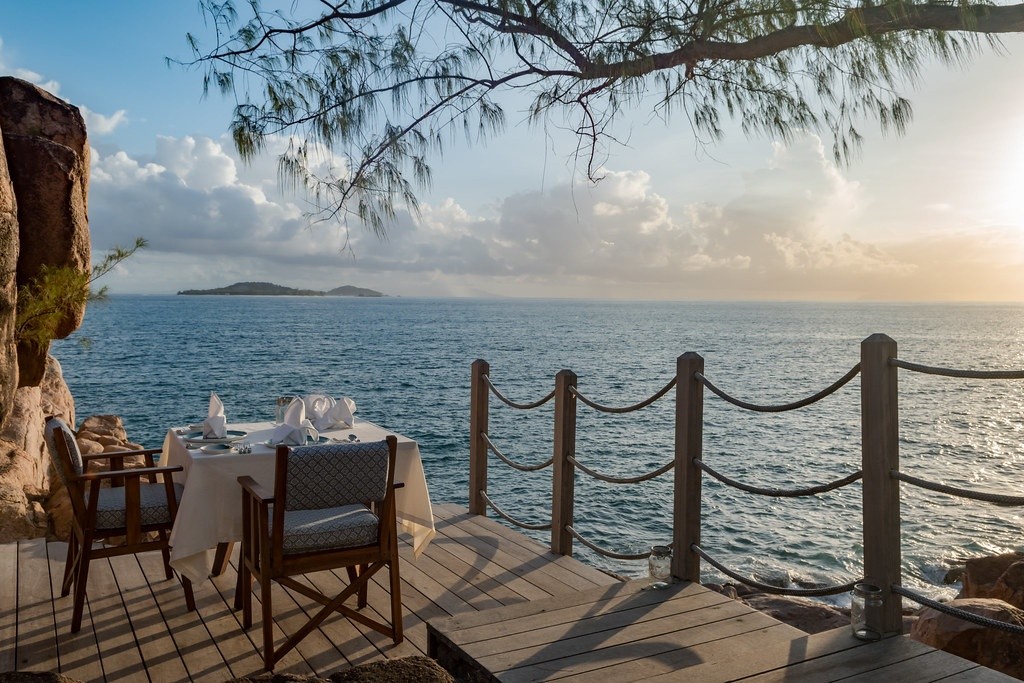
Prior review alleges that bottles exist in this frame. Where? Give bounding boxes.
[649,546,672,588]
[276,397,288,424]
[851,583,883,640]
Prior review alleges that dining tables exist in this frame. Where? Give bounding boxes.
[157,416,436,610]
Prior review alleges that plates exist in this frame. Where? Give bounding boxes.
[183,430,248,442]
[199,443,235,454]
[264,435,332,451]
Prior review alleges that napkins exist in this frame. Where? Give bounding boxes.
[270,395,319,445]
[202,390,228,439]
[303,394,356,429]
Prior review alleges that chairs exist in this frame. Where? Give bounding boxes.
[237,436,403,670]
[42,415,196,635]
